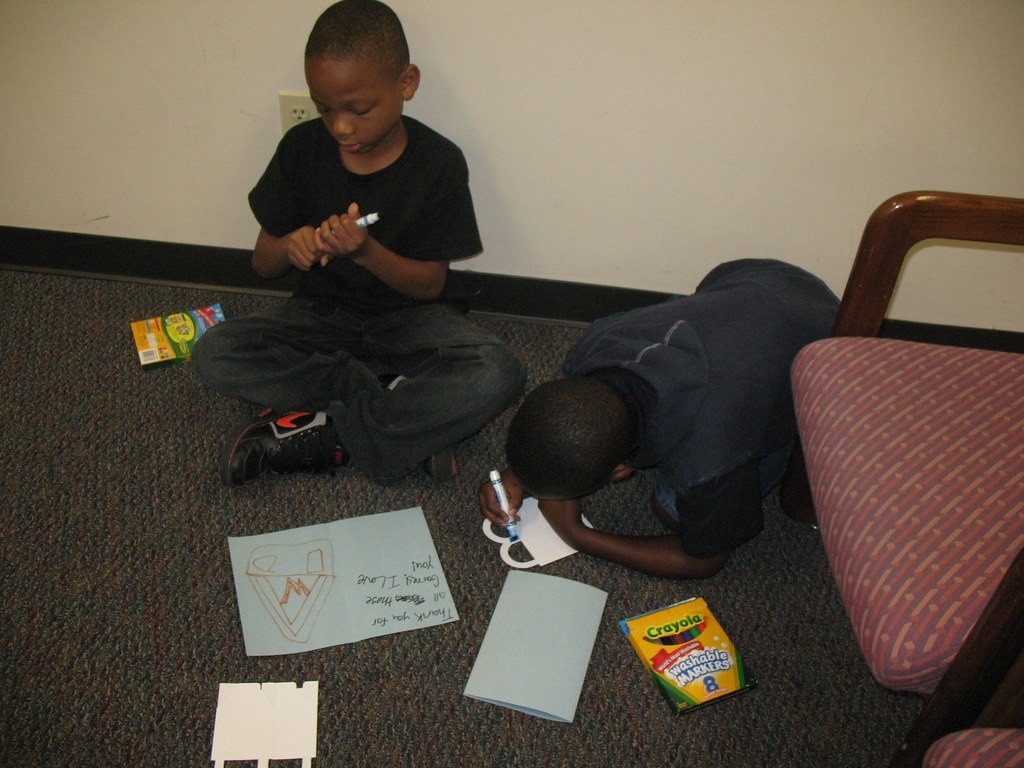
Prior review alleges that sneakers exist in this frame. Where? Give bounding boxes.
[220,405,345,486]
[427,445,457,479]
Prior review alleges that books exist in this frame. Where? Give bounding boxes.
[130,302,226,368]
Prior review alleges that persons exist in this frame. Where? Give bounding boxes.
[189,0,526,484]
[478,257,841,583]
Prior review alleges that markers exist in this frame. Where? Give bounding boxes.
[320,210,383,243]
[489,470,517,540]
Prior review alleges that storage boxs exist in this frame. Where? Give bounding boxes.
[619,597,759,719]
[130,303,225,371]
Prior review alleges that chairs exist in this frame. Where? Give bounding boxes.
[778,190,1024,768]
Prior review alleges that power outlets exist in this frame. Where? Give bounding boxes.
[279,92,318,137]
